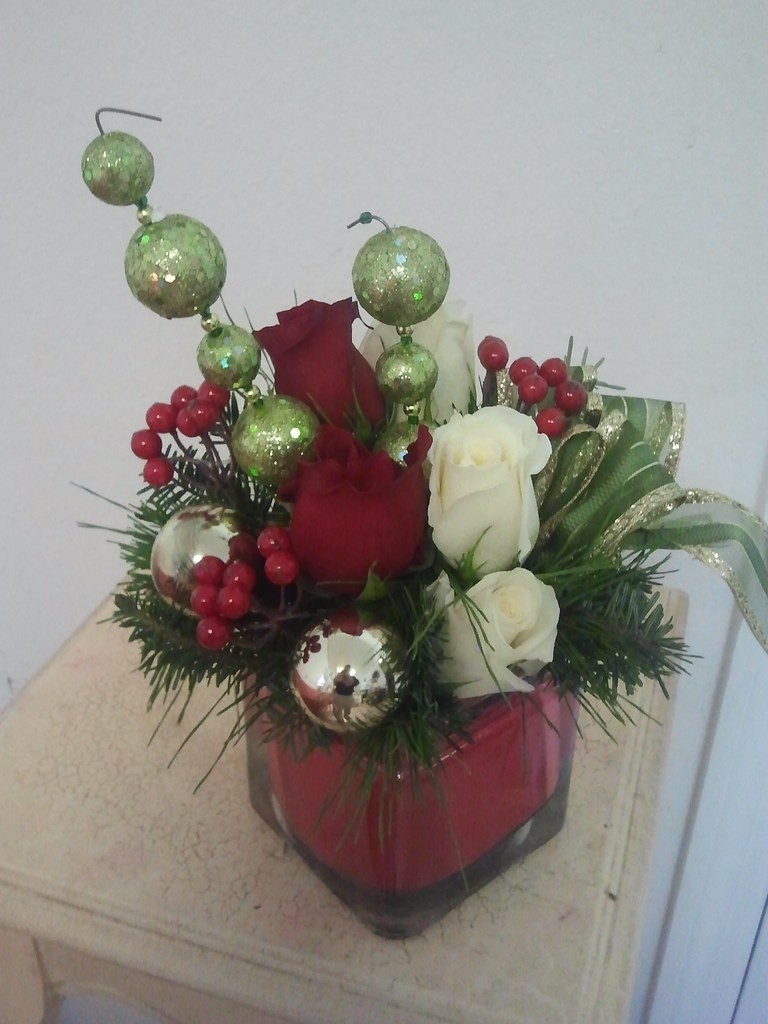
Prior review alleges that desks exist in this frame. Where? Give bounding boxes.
[0,563,689,1024]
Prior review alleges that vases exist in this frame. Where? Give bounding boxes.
[244,662,582,939]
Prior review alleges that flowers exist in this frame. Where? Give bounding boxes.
[67,108,768,853]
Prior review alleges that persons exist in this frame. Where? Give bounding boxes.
[332,664,359,724]
